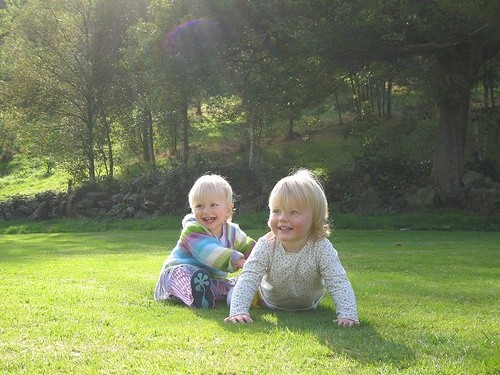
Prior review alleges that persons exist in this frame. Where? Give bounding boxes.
[152,172,257,310]
[224,166,362,327]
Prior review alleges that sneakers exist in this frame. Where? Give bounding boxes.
[191,268,214,311]
